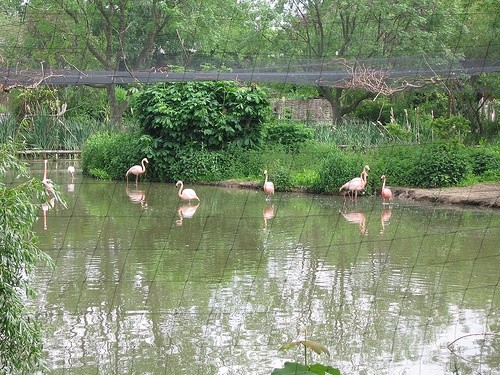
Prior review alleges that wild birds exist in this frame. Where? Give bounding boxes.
[380,175,394,203]
[67,166,78,182]
[263,170,274,200]
[338,165,371,202]
[175,180,201,202]
[35,159,63,199]
[125,158,149,191]
